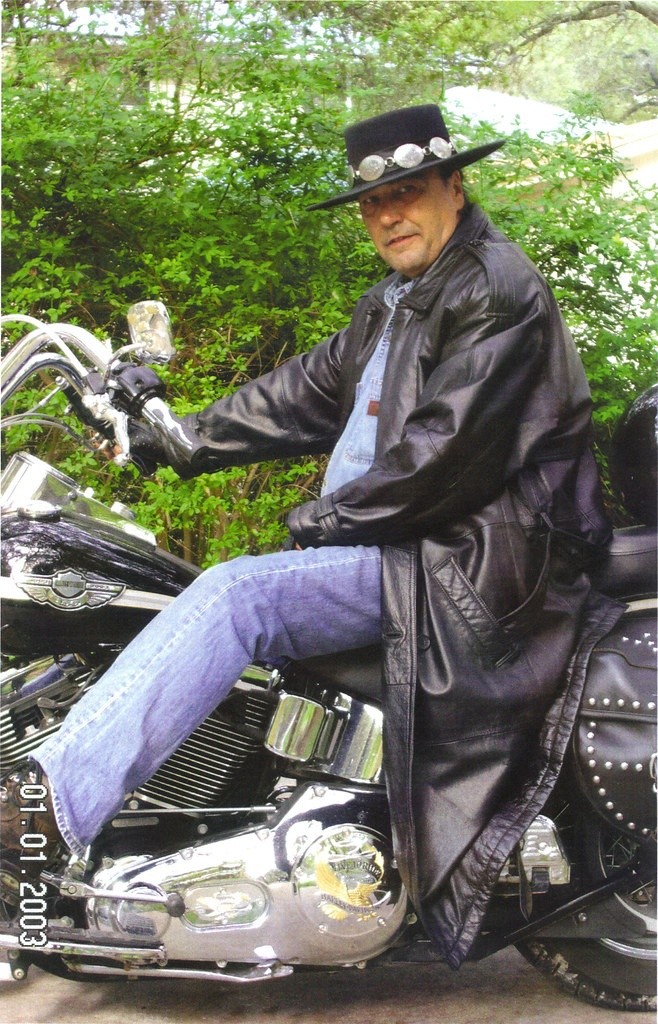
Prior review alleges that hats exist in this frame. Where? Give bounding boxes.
[304,104,506,211]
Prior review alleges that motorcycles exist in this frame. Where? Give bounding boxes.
[0,299,658,1013]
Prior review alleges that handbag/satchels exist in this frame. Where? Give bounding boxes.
[573,607,657,847]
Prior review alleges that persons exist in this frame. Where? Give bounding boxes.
[1,105,629,888]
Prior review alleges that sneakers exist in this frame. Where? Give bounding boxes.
[0,757,64,886]
